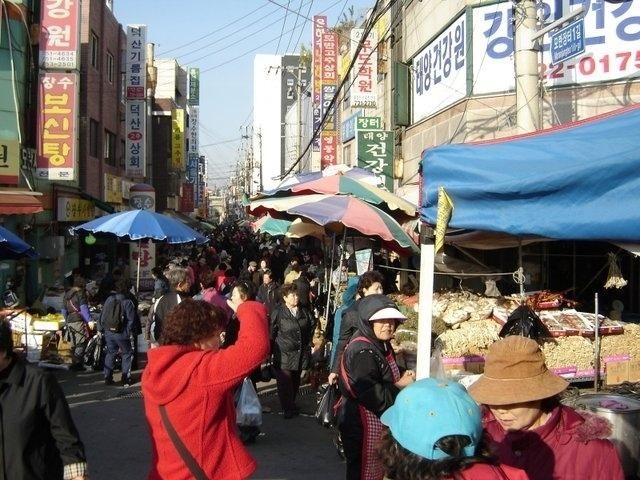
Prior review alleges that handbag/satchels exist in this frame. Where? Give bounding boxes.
[257,358,279,382]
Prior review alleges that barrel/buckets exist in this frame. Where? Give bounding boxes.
[137,322,148,354]
[559,393,640,480]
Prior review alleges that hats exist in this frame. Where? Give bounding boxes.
[467,334,571,408]
[379,377,483,460]
[368,306,408,322]
[249,261,257,267]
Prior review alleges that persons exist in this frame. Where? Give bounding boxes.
[0,310,88,480]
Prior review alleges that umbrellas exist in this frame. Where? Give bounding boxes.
[1,225,41,260]
[251,217,334,288]
[245,195,419,311]
[248,173,419,329]
[72,205,211,292]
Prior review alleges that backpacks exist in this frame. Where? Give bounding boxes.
[105,295,131,334]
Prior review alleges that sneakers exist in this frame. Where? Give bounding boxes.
[237,407,300,445]
[73,359,136,386]
